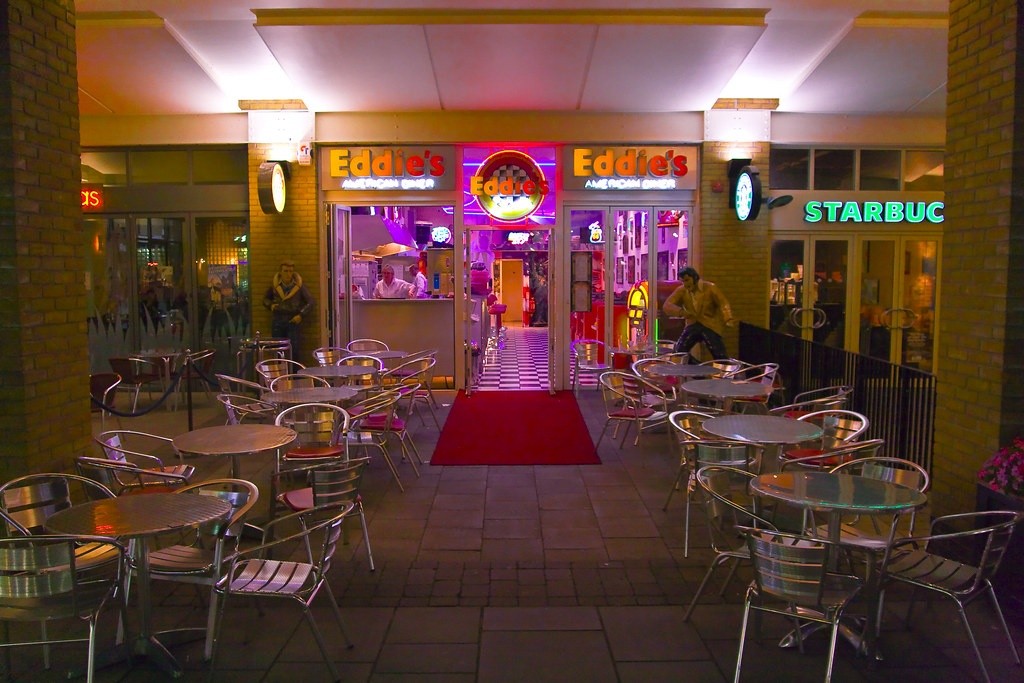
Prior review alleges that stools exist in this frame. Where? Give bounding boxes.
[489,304,507,343]
[486,308,506,350]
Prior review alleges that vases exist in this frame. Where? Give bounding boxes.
[976,482,1024,592]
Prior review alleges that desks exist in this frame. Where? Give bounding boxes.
[701,414,825,528]
[645,363,717,410]
[174,425,298,546]
[262,387,359,441]
[343,350,409,368]
[43,493,233,683]
[297,365,379,386]
[130,351,189,411]
[749,471,927,662]
[680,380,774,416]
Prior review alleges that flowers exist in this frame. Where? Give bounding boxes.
[978,437,1024,491]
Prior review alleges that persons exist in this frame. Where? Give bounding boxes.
[262,261,312,376]
[409,264,427,298]
[663,266,735,367]
[372,264,417,299]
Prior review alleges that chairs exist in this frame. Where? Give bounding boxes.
[662,411,716,511]
[594,371,677,456]
[768,385,854,422]
[570,339,615,399]
[614,358,677,446]
[722,362,779,415]
[698,359,742,382]
[732,525,883,683]
[802,456,929,667]
[875,511,1021,683]
[679,440,767,559]
[684,464,806,656]
[0,338,441,683]
[769,438,885,527]
[771,410,870,525]
[655,352,689,366]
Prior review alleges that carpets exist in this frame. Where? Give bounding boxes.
[429,389,604,466]
